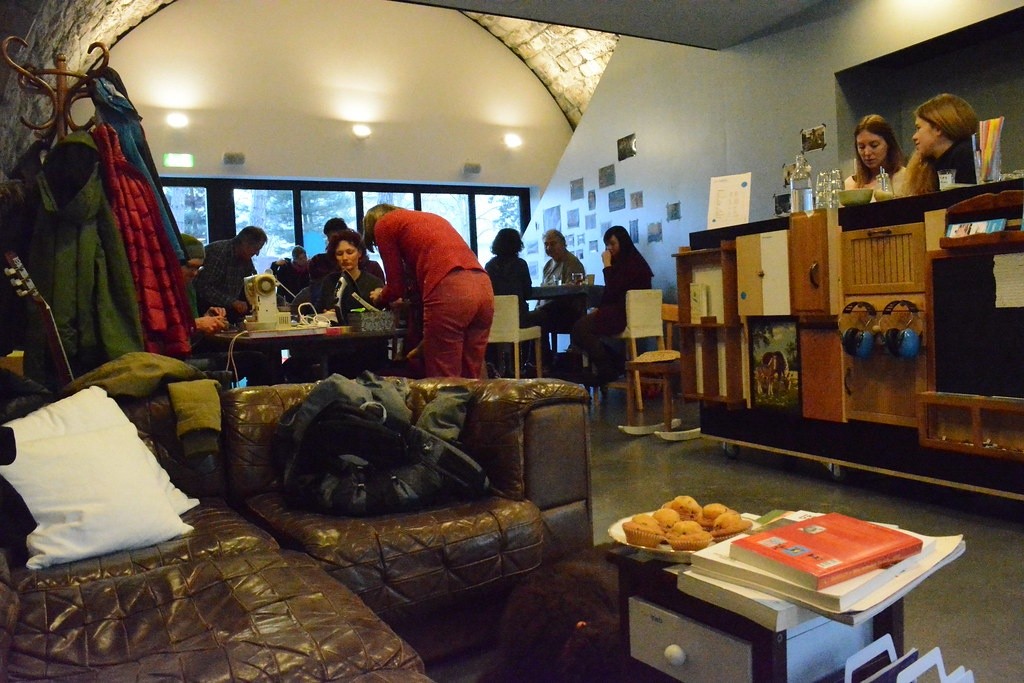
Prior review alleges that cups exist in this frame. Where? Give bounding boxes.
[974,150,1000,185]
[572,273,583,285]
[937,169,956,190]
[554,274,562,286]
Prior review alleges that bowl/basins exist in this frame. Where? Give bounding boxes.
[838,188,873,206]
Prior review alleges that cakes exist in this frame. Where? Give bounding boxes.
[625,495,753,550]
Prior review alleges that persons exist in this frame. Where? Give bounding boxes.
[177,218,385,387]
[361,204,496,379]
[852,113,908,202]
[905,93,1001,394]
[570,225,653,378]
[485,228,551,362]
[531,229,587,358]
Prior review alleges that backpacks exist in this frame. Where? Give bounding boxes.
[277,399,492,521]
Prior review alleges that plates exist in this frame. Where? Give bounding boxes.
[607,511,762,563]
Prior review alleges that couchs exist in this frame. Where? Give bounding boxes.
[0,377,595,683]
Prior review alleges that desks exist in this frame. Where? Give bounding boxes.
[207,326,410,384]
[527,283,587,355]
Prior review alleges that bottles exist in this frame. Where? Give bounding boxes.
[815,170,844,209]
[874,173,894,201]
[790,154,813,212]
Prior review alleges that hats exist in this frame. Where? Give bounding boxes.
[181,233,206,260]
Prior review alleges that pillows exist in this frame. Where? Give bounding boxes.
[0,386,201,517]
[0,423,196,571]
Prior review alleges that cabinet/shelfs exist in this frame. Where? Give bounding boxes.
[790,209,831,316]
[844,309,927,428]
[842,218,925,298]
[605,543,906,683]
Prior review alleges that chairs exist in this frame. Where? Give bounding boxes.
[485,294,544,379]
[619,290,703,441]
